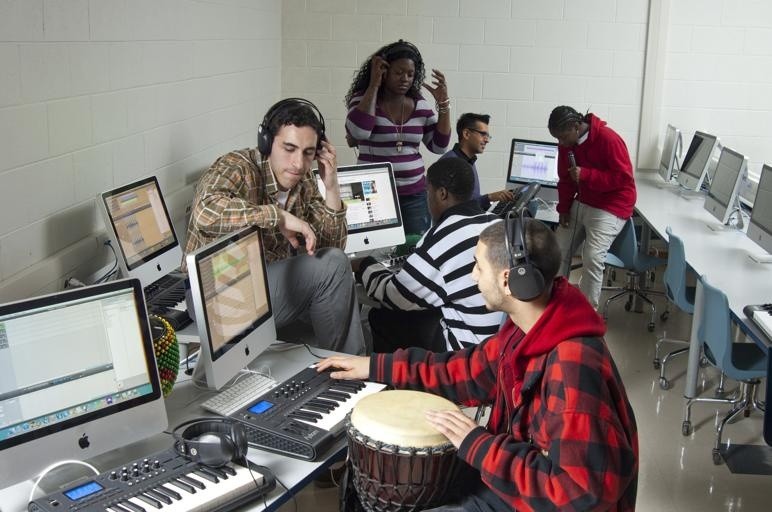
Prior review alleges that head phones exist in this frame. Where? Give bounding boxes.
[505,207,546,301]
[380,45,418,81]
[172,417,248,468]
[258,97,325,155]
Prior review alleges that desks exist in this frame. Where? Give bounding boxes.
[628,169,772,350]
[2,271,389,512]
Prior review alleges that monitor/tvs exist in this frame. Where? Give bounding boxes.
[186,225,277,391]
[659,122,772,263]
[312,162,406,254]
[0,277,169,490]
[97,175,183,288]
[507,139,560,188]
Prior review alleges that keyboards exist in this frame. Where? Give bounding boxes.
[199,374,281,416]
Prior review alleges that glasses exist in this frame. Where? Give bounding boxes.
[466,127,489,138]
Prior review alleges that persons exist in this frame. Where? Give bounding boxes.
[181,97,366,357]
[344,39,451,236]
[438,113,514,211]
[351,157,505,354]
[316,207,639,512]
[548,106,637,312]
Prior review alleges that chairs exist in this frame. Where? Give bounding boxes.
[598,217,669,332]
[682,273,770,465]
[654,224,698,389]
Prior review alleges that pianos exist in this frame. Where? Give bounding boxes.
[486,182,541,219]
[28,436,275,511]
[143,276,189,329]
[224,361,390,459]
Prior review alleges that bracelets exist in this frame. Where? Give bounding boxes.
[434,97,450,113]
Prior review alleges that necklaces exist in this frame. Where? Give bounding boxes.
[386,97,404,153]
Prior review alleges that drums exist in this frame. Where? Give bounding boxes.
[345,389,464,511]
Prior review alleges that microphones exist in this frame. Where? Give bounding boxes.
[568,151,579,185]
